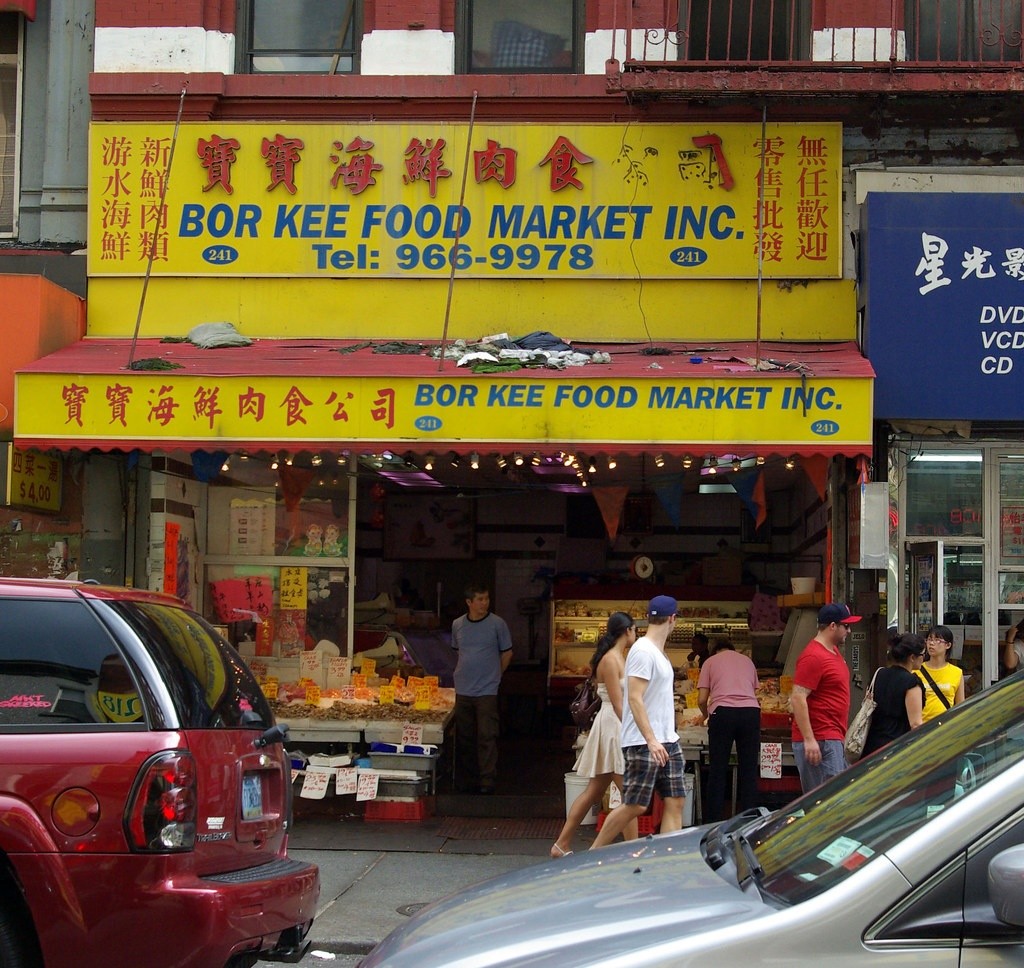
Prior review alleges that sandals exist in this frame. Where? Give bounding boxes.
[548,841,574,858]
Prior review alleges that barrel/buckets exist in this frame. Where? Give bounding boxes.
[791,577,815,595]
[564,772,601,825]
[682,774,695,827]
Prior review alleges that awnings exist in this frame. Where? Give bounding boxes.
[12,336,875,463]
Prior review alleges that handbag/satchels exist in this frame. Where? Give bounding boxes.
[843,666,883,760]
[570,682,602,728]
[485,20,566,75]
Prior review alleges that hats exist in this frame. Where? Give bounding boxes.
[816,602,863,623]
[647,595,678,614]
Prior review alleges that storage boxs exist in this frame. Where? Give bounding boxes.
[762,712,789,728]
[596,809,655,834]
[377,779,430,797]
[757,775,802,791]
[363,799,426,822]
[367,752,440,769]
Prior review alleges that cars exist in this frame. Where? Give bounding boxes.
[358,664,1023,967]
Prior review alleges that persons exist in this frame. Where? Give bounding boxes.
[548,595,1023,859]
[451,583,513,794]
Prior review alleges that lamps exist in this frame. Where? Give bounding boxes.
[223,451,617,487]
[655,452,796,472]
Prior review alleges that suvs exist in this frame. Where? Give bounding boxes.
[0,574,323,968]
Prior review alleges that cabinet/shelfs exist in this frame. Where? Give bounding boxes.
[547,583,756,698]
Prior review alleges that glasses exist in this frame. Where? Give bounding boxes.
[839,621,853,629]
[924,637,952,646]
[914,651,930,660]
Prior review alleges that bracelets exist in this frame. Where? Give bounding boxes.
[1005,640,1014,644]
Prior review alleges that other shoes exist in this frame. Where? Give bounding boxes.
[448,782,471,793]
[478,777,497,795]
